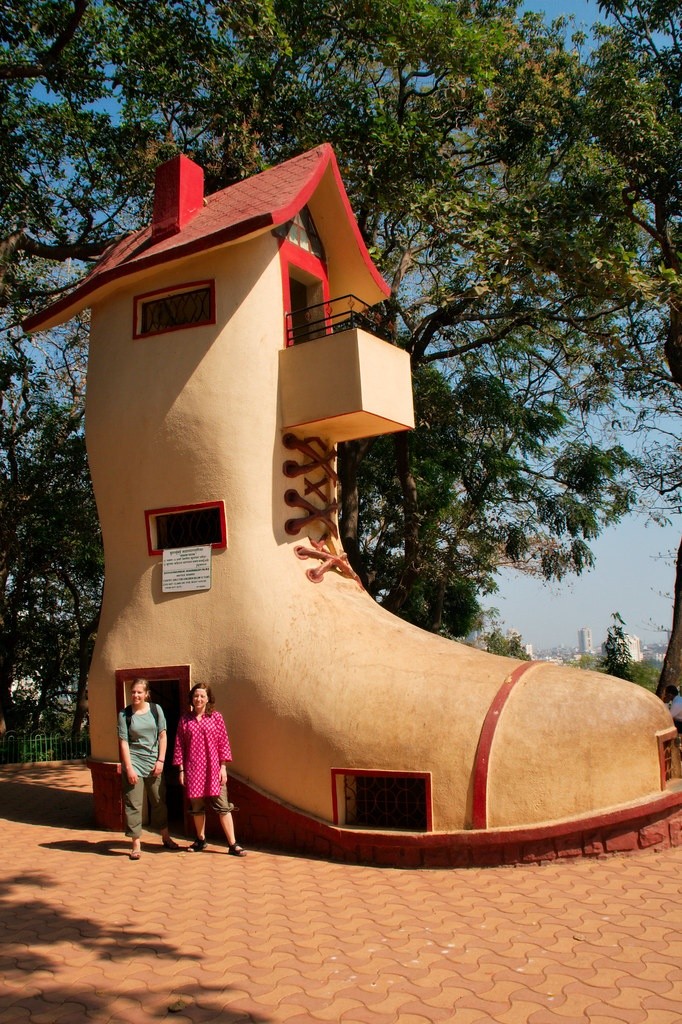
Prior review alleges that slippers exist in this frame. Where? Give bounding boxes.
[163,839,179,849]
[131,849,140,860]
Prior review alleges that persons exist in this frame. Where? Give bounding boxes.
[173,683,247,857]
[664,685,682,734]
[117,678,178,859]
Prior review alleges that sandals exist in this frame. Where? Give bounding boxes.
[228,842,247,856]
[187,839,206,852]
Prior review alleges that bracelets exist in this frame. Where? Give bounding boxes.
[179,770,184,772]
[158,760,164,763]
